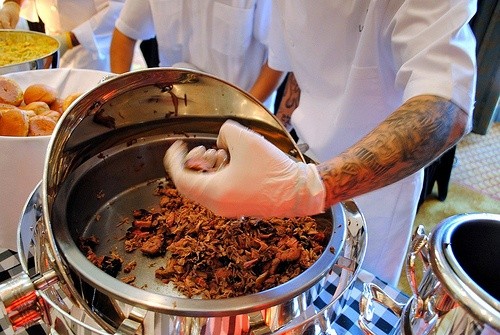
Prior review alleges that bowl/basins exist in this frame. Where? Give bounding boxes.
[0,29,61,75]
[1,68,120,252]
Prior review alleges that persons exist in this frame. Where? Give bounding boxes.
[0,0,149,76]
[109,1,287,116]
[162,0,477,291]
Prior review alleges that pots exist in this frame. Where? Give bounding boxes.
[359,212,500,335]
